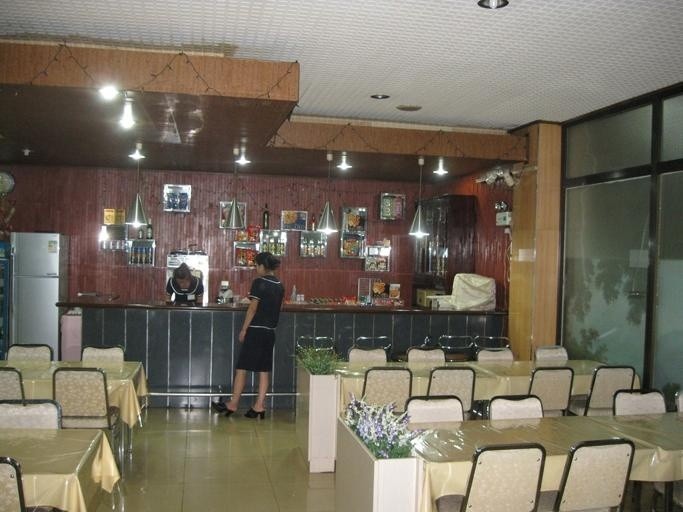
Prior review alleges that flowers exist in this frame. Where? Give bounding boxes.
[338,394,417,458]
[293,340,340,375]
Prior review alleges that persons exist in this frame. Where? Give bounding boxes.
[163,262,204,307]
[211,251,286,420]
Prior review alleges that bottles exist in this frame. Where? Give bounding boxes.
[137,227,143,238]
[675,389,682,420]
[311,213,316,230]
[101,240,125,251]
[146,218,152,239]
[130,247,152,265]
[262,203,268,229]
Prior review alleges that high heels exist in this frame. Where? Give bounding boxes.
[210,399,234,417]
[244,408,266,420]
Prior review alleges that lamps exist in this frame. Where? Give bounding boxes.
[219,149,244,232]
[493,201,512,227]
[115,90,136,131]
[336,151,354,171]
[234,137,251,166]
[130,140,145,161]
[404,160,429,240]
[125,142,149,228]
[431,158,449,176]
[316,154,338,234]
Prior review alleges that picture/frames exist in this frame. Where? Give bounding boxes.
[301,232,330,258]
[365,245,389,273]
[259,230,287,257]
[280,210,308,232]
[219,202,246,230]
[380,193,407,223]
[341,206,367,263]
[163,184,191,212]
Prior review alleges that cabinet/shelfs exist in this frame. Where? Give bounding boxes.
[418,195,475,296]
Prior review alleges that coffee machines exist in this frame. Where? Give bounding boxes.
[217,280,234,305]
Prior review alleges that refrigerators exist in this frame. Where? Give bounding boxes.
[10,231,70,363]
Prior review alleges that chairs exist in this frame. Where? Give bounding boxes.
[80,346,125,364]
[488,395,545,420]
[404,397,463,422]
[651,480,683,512]
[1,400,62,431]
[348,348,386,365]
[52,367,118,458]
[0,368,25,403]
[459,444,546,512]
[6,344,53,362]
[566,365,635,416]
[426,367,475,419]
[552,439,635,511]
[612,389,668,501]
[526,367,573,417]
[535,346,568,361]
[675,391,683,412]
[477,348,513,361]
[0,457,27,512]
[407,347,446,364]
[361,367,412,415]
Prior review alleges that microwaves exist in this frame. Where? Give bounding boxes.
[416,289,446,308]
